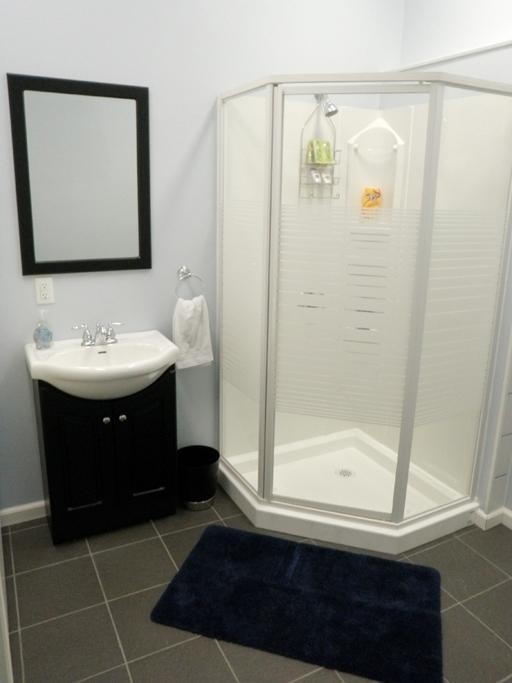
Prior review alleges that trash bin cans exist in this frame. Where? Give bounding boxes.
[178,444,220,511]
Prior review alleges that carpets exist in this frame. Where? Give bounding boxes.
[151,524,444,682]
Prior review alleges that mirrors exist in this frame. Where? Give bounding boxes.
[6,72,153,275]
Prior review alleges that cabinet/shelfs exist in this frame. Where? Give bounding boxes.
[32,365,176,544]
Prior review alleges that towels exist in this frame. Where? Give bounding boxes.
[171,296,215,367]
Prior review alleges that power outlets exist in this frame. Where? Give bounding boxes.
[34,277,55,304]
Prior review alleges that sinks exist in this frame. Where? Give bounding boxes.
[24,330,180,400]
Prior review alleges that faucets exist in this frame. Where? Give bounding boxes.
[71,318,126,347]
